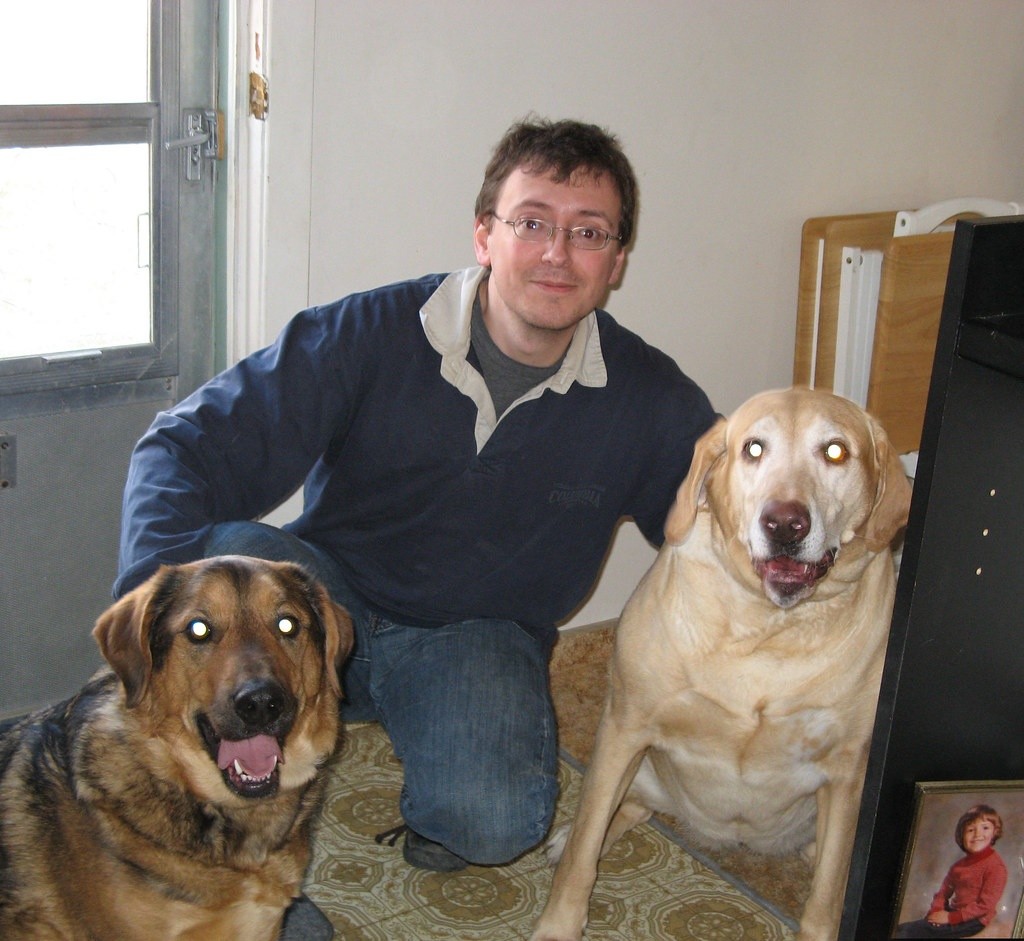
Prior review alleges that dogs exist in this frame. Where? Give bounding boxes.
[1,555,356,941]
[526,386,913,941]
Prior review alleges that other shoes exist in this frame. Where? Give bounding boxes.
[374,822,469,872]
[281,890,336,941]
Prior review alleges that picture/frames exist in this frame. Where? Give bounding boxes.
[887,781,1024,939]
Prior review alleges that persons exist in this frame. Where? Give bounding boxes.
[895,803,1008,939]
[110,120,719,941]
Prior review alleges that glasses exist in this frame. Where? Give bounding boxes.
[488,211,624,250]
